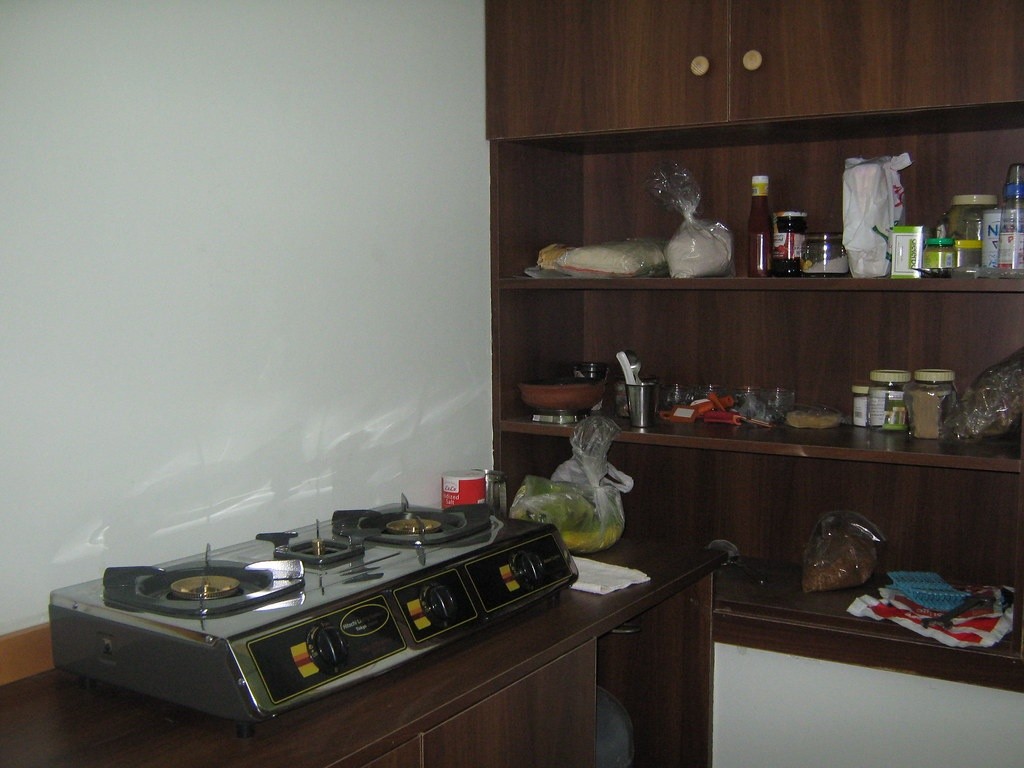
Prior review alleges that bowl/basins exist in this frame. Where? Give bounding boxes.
[518,377,607,413]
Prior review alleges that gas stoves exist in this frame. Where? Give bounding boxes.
[47,491,579,742]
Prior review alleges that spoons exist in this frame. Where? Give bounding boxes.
[622,350,642,384]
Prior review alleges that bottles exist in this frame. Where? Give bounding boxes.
[998,162,1024,279]
[747,175,775,278]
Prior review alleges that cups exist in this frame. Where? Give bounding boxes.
[657,383,796,423]
[626,383,658,427]
[578,361,607,381]
[471,468,507,517]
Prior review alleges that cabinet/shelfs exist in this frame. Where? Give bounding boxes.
[0,543,728,768]
[484,0,1024,692]
